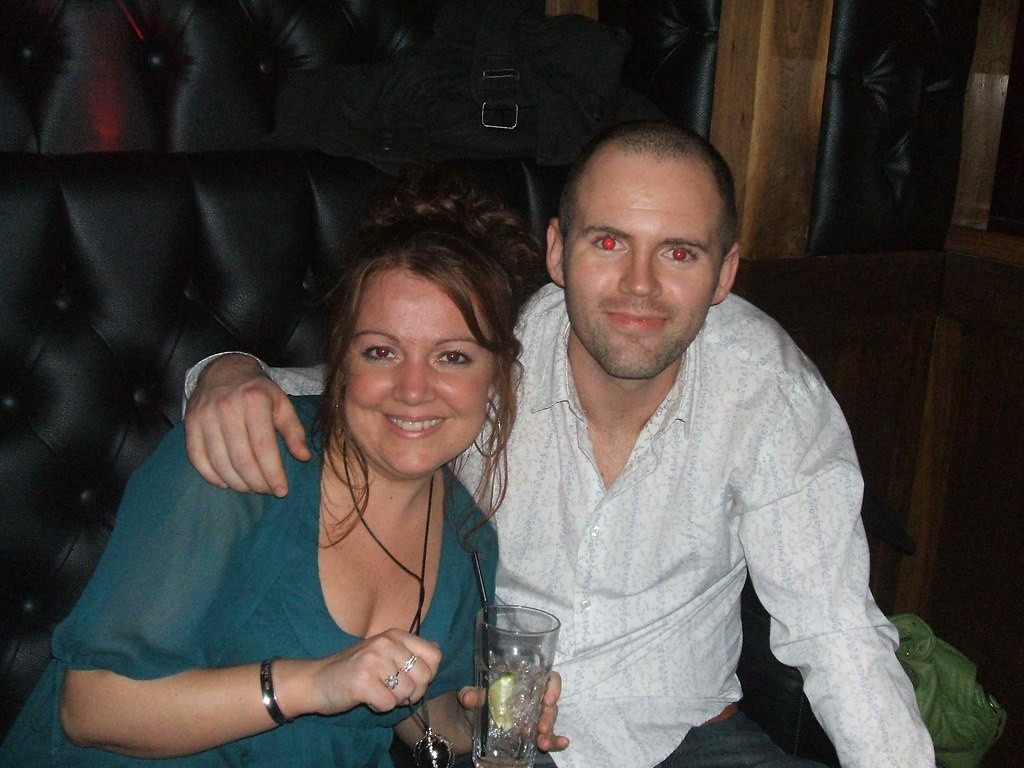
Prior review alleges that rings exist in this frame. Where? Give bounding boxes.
[400,654,417,673]
[384,671,401,689]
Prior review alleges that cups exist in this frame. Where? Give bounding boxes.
[472,605,561,768]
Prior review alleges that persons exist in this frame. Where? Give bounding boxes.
[0,165,551,768]
[181,114,937,768]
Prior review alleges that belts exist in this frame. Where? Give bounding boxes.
[704,703,738,726]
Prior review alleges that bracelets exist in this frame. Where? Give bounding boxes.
[261,655,296,725]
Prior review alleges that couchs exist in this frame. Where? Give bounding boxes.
[0,0,918,759]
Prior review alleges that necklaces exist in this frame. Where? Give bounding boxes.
[344,430,454,768]
